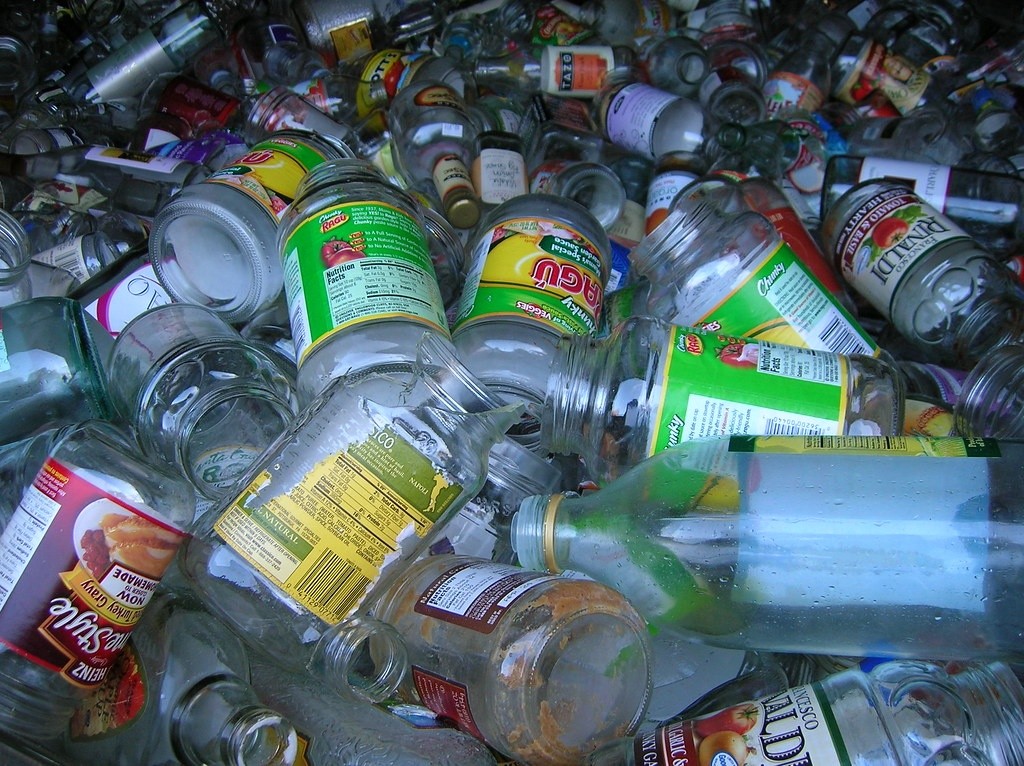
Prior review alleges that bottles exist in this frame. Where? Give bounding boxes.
[0,1,1024,765]
